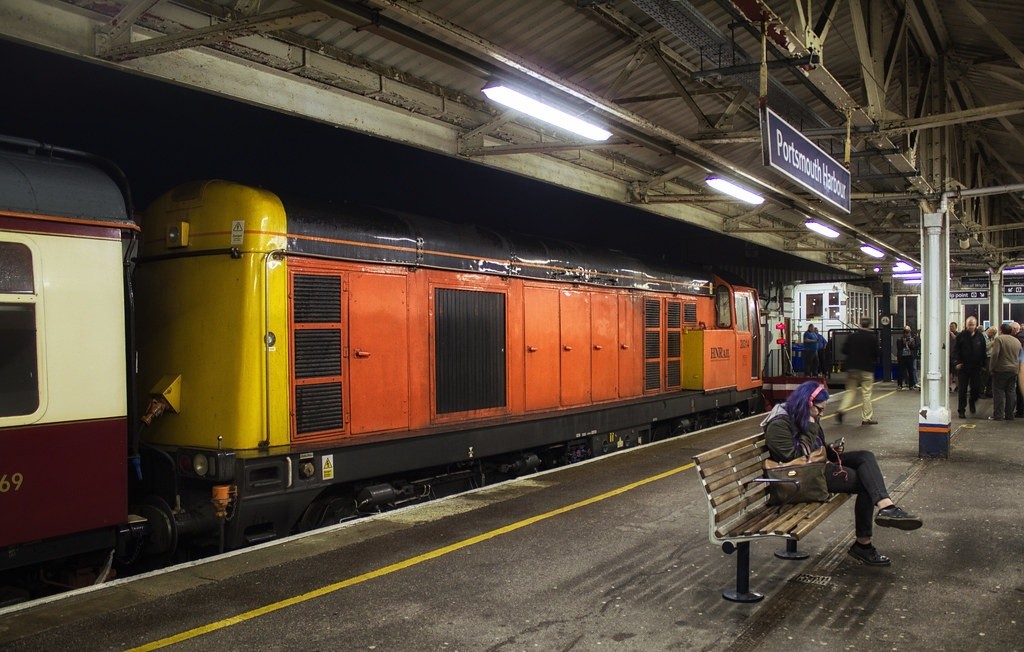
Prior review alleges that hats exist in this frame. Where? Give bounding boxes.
[904,325,911,331]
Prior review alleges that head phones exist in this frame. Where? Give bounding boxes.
[808,386,824,408]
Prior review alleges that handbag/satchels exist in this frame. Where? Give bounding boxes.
[764,443,829,504]
[902,342,912,356]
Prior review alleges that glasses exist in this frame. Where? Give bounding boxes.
[814,405,824,414]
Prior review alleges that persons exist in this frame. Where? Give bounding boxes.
[803,324,828,378]
[896,316,1024,420]
[760,382,923,567]
[835,319,881,424]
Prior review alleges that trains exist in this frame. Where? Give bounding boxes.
[0,131,767,597]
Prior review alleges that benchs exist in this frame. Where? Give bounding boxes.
[691,432,855,603]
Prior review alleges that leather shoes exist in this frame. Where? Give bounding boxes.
[847,544,891,567]
[875,508,923,531]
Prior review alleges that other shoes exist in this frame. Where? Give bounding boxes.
[949,386,959,394]
[805,373,822,379]
[834,412,842,425]
[959,411,965,419]
[862,421,878,425]
[969,400,976,413]
[1014,411,1024,418]
[988,416,1013,421]
[979,393,993,399]
[896,384,920,391]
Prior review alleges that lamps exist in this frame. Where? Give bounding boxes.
[480,81,921,284]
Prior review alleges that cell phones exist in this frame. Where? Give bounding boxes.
[833,437,844,447]
